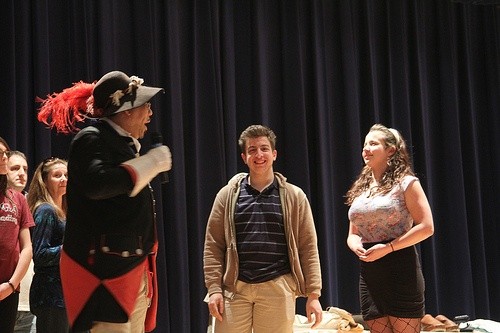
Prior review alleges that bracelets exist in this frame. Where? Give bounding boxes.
[389,242,394,252]
[6,280,16,293]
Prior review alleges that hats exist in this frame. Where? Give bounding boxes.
[36,71,163,135]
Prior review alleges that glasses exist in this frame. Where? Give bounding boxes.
[41,156,64,172]
[140,103,151,110]
[0,148,11,156]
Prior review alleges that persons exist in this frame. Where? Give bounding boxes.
[0,137,33,333]
[6,150,35,332]
[202,124,322,333]
[347,124,434,333]
[58,73,170,333]
[29,157,68,333]
